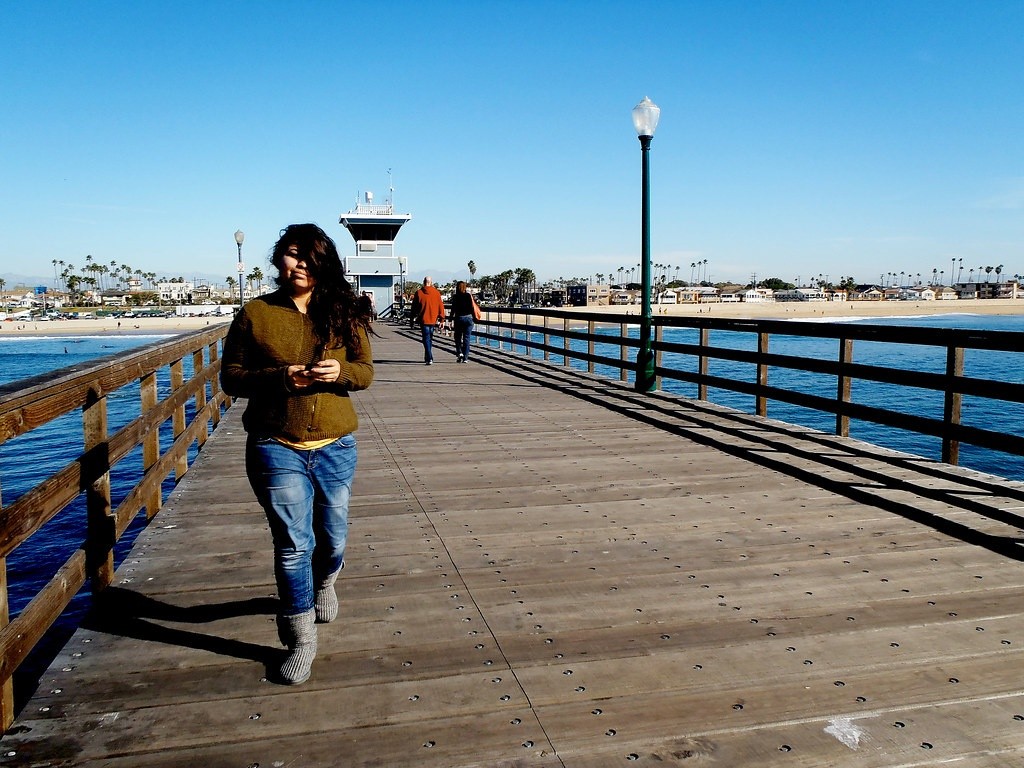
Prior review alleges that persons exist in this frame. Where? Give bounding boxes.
[18,325,21,330]
[206,321,210,325]
[626,303,855,313]
[22,324,26,328]
[34,323,37,330]
[409,276,445,365]
[447,281,474,363]
[218,223,376,686]
[118,322,121,327]
[394,292,414,301]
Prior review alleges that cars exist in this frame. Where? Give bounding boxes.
[18,310,229,321]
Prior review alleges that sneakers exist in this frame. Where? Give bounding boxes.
[426,359,432,365]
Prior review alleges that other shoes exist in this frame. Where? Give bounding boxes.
[457,353,463,363]
[461,361,467,363]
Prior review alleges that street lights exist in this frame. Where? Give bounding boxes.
[630,94,661,392]
[234,228,246,307]
[397,256,404,314]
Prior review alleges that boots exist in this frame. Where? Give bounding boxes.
[314,561,345,622]
[276,606,318,687]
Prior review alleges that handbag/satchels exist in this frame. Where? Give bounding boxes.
[469,293,481,320]
